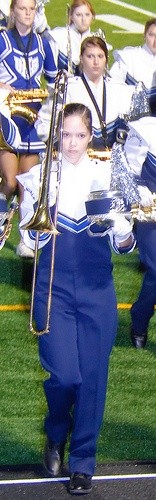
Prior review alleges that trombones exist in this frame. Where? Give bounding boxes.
[20,68,71,335]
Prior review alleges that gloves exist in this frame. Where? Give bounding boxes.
[106,210,134,242]
[137,185,155,207]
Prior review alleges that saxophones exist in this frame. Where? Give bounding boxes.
[0,194,19,243]
[0,85,52,126]
[129,193,156,219]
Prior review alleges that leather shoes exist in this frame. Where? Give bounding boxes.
[132,332,146,350]
[69,471,91,494]
[43,440,63,475]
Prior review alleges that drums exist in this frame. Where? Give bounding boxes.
[87,189,129,222]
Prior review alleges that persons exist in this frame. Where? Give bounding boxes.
[0,0,57,257]
[50,0,112,75]
[110,18,156,106]
[124,95,156,349]
[34,36,133,149]
[18,102,136,492]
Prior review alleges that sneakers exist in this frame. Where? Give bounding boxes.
[15,242,34,259]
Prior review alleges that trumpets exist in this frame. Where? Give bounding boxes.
[38,148,114,160]
